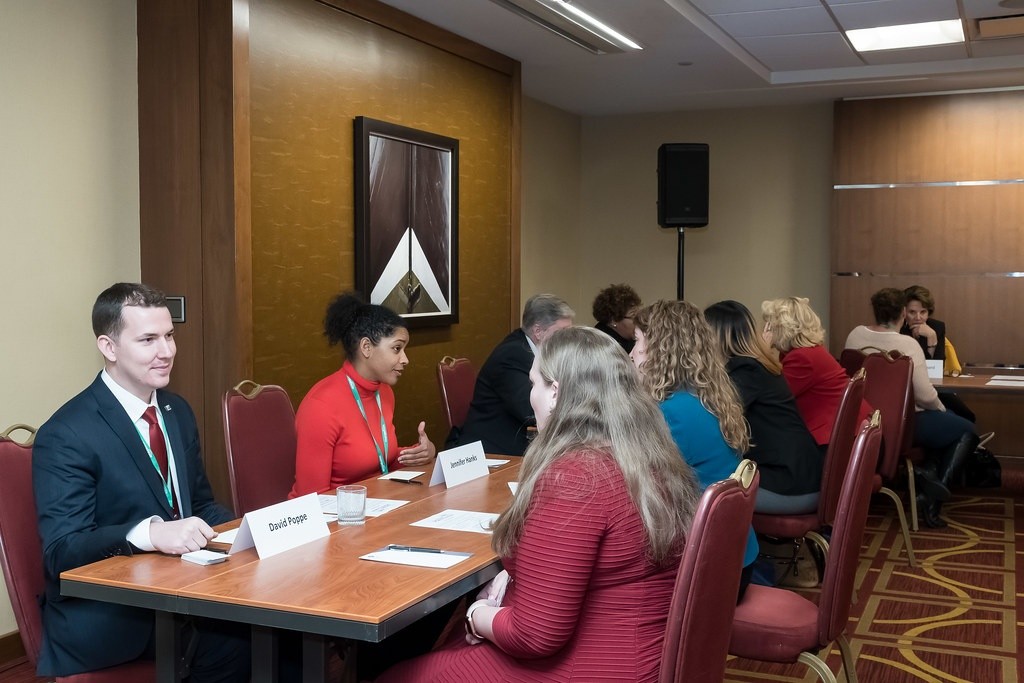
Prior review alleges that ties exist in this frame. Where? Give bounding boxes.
[140,406,180,517]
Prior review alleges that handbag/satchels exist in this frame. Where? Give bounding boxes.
[949,432,1002,488]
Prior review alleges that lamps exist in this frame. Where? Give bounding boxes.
[490,0,644,54]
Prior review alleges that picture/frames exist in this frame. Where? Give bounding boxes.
[353,116,459,326]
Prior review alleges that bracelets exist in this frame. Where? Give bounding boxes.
[465,602,490,640]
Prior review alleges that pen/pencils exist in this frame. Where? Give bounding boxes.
[390,479,422,485]
[201,548,226,553]
[389,546,444,553]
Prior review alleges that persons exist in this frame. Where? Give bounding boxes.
[378,326,703,683]
[630,284,1003,606]
[32,283,285,681]
[295,293,436,681]
[462,284,641,454]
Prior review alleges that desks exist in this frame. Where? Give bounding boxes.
[58,454,523,683]
[930,375,1024,457]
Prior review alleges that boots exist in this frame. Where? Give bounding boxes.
[906,431,980,528]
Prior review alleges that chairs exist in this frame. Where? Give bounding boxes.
[221,380,295,519]
[436,356,473,431]
[659,346,923,683]
[0,424,156,683]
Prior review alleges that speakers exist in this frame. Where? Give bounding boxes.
[656,143,709,229]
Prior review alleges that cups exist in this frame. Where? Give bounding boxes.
[335,484,367,526]
